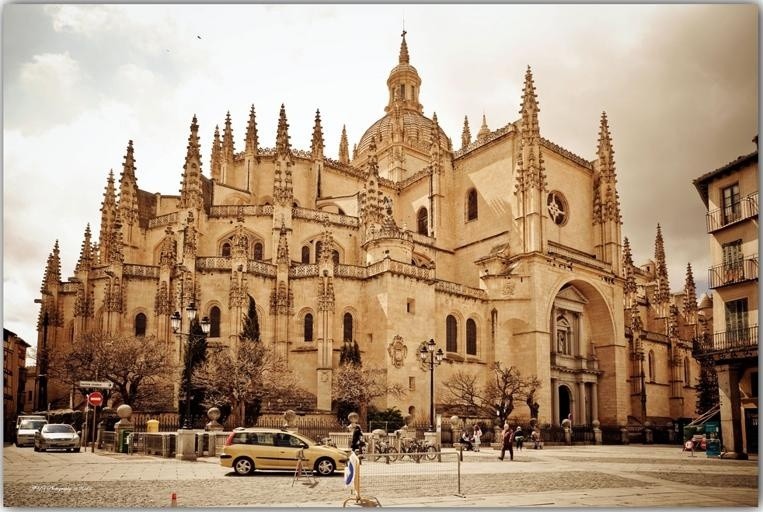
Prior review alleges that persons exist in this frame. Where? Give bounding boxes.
[470,425,482,452]
[496,424,515,461]
[513,425,524,452]
[281,434,291,447]
[246,433,258,445]
[350,424,366,452]
[567,409,573,434]
[461,431,473,451]
[95,417,104,449]
[530,423,540,450]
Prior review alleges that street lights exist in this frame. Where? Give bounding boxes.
[170,303,211,430]
[419,339,443,433]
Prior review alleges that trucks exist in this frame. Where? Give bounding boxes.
[15,416,46,438]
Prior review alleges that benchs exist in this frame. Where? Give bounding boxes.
[491,443,503,449]
[524,442,544,449]
[453,443,475,451]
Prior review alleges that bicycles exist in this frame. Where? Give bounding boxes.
[352,438,437,463]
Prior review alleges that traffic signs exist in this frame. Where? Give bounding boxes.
[79,381,113,390]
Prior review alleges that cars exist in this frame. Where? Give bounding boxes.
[15,420,48,447]
[34,423,82,452]
[219,427,348,477]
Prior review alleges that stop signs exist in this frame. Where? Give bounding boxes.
[700,441,707,450]
[87,392,103,407]
